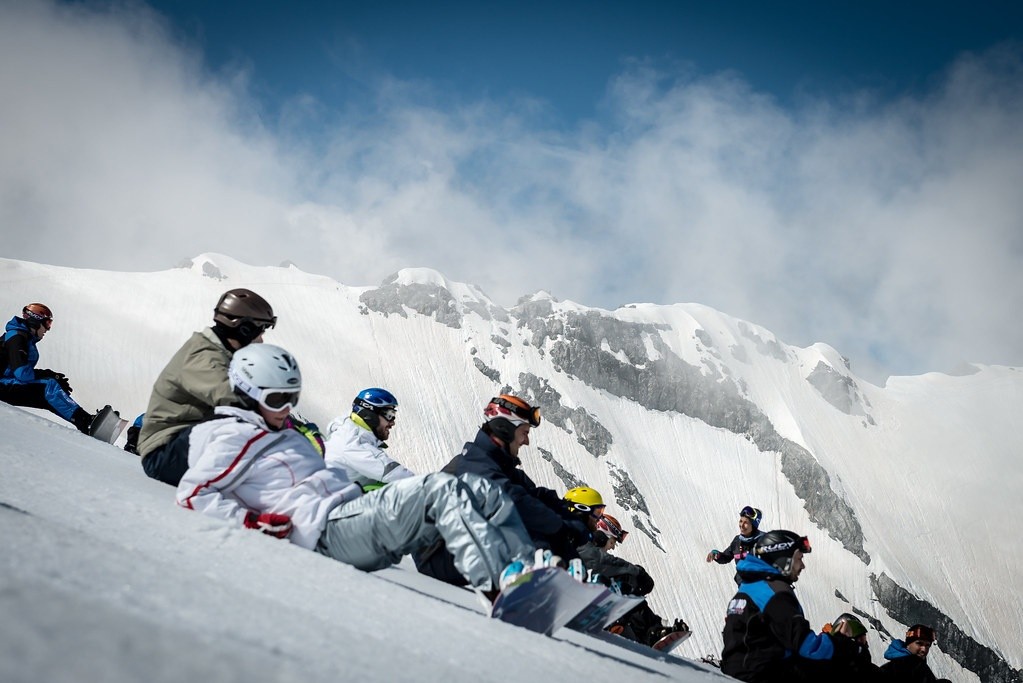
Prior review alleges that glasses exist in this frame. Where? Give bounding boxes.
[529,405,541,426]
[617,530,629,544]
[42,317,53,330]
[259,388,302,411]
[739,506,758,523]
[587,504,606,519]
[797,536,811,554]
[381,407,397,421]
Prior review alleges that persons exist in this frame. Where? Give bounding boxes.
[720,529,953,683]
[706,506,767,665]
[124,289,692,652]
[0,303,120,436]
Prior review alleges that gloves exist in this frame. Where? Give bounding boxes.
[830,632,871,665]
[561,513,590,546]
[244,511,296,540]
[34,369,73,395]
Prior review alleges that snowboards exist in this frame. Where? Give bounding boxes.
[491,566,607,635]
[653,631,692,654]
[564,587,645,632]
[89,405,129,446]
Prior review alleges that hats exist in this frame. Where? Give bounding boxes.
[740,508,762,529]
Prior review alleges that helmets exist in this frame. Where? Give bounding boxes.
[831,613,868,639]
[755,530,801,579]
[22,303,54,321]
[563,486,604,515]
[596,514,622,538]
[228,342,302,393]
[906,624,934,647]
[213,288,277,329]
[350,388,398,428]
[482,394,532,428]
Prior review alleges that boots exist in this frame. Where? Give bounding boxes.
[71,406,120,436]
